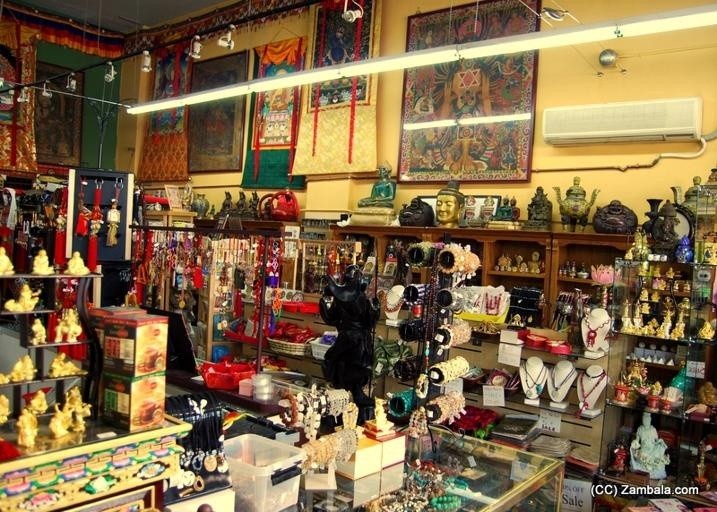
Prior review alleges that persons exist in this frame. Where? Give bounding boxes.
[436,182,465,230]
[357,162,395,207]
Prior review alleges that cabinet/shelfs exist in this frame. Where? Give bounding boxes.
[1,267,106,423]
[252,223,717,511]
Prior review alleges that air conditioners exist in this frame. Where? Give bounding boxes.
[542,96,704,149]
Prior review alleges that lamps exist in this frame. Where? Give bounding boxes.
[339,1,365,23]
[13,24,235,104]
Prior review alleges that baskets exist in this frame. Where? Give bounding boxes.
[266,337,312,357]
[309,336,335,361]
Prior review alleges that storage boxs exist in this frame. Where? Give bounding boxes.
[85,305,170,433]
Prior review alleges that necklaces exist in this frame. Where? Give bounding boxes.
[524,358,547,399]
[580,368,607,416]
[551,359,576,399]
[389,242,480,439]
[384,291,404,313]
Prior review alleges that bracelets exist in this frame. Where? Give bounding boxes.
[367,458,468,512]
[277,388,358,471]
